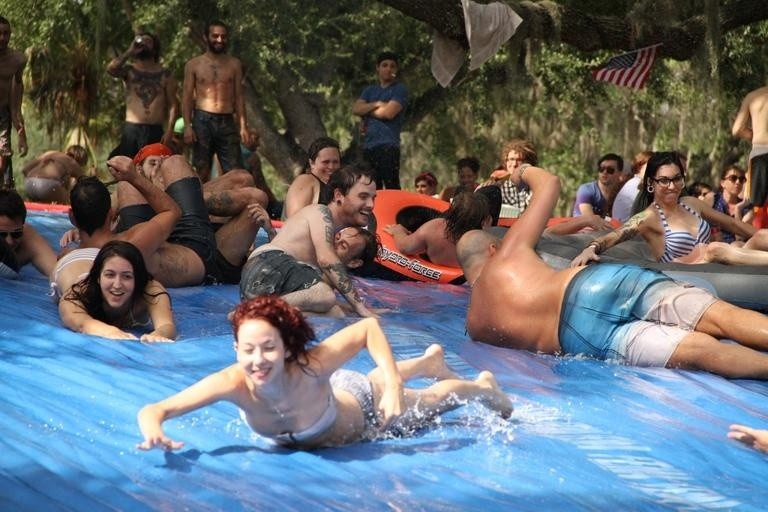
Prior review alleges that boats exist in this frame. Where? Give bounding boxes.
[478,223,767,312]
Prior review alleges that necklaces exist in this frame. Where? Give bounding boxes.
[471,275,479,288]
[257,375,284,418]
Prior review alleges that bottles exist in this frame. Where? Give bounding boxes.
[709,224,722,242]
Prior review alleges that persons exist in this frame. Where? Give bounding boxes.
[2,189,57,278]
[571,151,767,267]
[415,170,439,198]
[228,161,399,322]
[44,239,177,342]
[0,14,29,190]
[573,154,624,219]
[607,151,652,225]
[107,32,178,160]
[57,143,275,289]
[279,136,341,222]
[727,424,766,448]
[352,51,408,190]
[23,145,98,205]
[456,163,766,381]
[181,19,249,184]
[383,191,493,268]
[473,139,615,236]
[246,203,382,274]
[732,86,768,218]
[686,165,767,248]
[439,157,480,204]
[138,295,513,449]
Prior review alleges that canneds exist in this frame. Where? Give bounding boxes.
[135,36,144,46]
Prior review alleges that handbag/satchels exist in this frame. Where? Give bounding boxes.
[372,145,399,172]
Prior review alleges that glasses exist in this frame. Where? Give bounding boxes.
[1,232,23,240]
[728,175,746,183]
[650,175,684,187]
[599,166,614,174]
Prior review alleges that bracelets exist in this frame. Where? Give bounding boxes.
[519,166,534,182]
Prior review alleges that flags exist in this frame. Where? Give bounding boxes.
[593,45,657,89]
